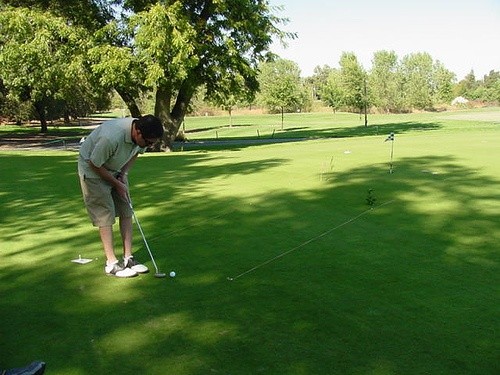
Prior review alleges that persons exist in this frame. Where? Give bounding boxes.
[77,114,165,277]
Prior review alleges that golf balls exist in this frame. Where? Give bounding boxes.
[170,271,176,277]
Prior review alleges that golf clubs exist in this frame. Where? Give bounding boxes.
[123,194,166,279]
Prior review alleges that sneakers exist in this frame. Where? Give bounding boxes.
[105,261,137,278]
[121,257,148,273]
